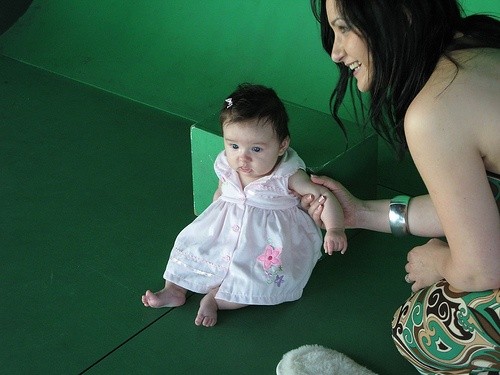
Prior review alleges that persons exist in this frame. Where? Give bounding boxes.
[300,0,500,375]
[142,82,348,327]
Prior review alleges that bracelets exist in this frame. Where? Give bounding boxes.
[387,194,412,238]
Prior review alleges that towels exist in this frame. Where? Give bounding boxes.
[276,344,379,375]
